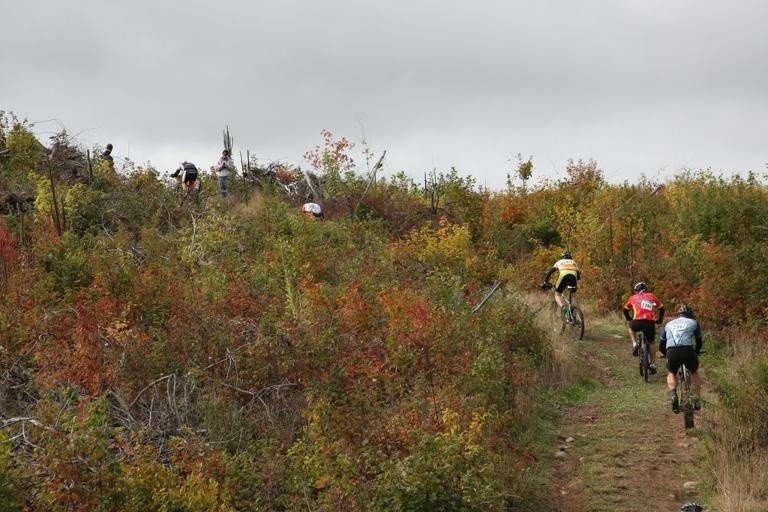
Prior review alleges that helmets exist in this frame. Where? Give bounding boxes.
[676,303,695,317]
[633,281,649,292]
[560,249,573,259]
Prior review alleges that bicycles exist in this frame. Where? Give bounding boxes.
[659,350,707,433]
[625,317,662,380]
[540,282,586,339]
[170,173,202,208]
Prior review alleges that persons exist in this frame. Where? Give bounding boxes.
[660,305,702,413]
[623,282,664,374]
[215,150,233,199]
[171,162,198,195]
[544,252,580,323]
[102,144,114,168]
[301,198,323,220]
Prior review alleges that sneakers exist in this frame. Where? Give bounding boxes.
[692,395,702,411]
[649,363,658,376]
[562,306,571,320]
[631,344,640,357]
[670,394,682,415]
